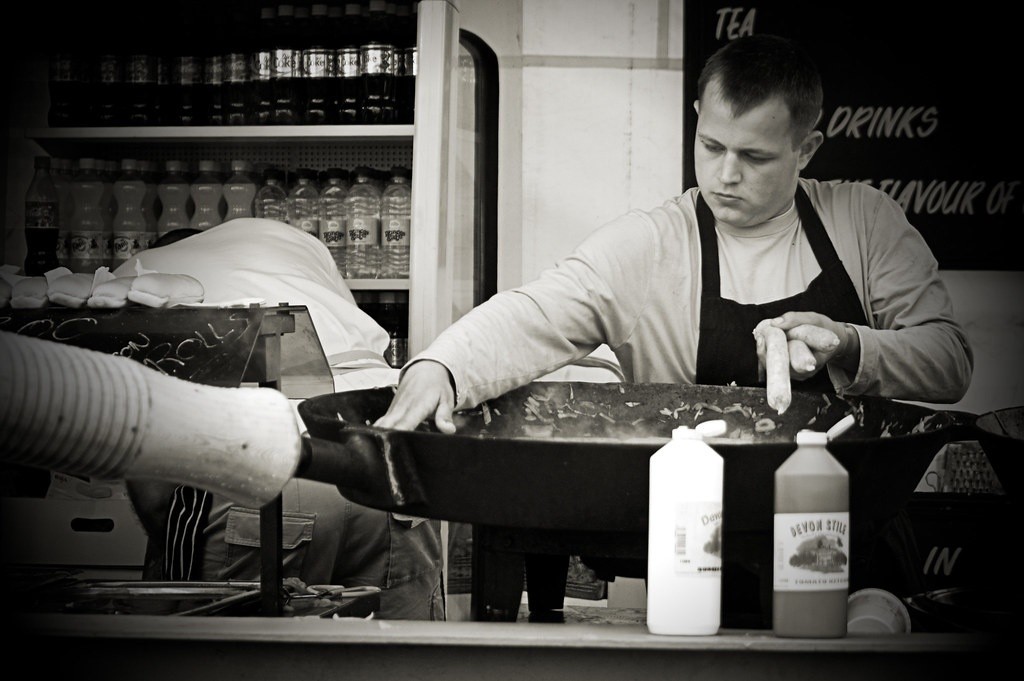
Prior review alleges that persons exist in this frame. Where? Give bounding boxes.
[111,217,448,622]
[373,34,975,436]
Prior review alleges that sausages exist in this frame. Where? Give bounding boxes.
[754,318,839,415]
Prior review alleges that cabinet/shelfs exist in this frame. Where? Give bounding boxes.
[0,0,460,368]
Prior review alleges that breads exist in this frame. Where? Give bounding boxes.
[0,271,204,309]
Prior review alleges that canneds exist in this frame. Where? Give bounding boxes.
[50,41,416,125]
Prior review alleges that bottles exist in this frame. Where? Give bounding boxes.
[47,0,417,127]
[23,153,411,280]
[646,426,724,635]
[773,430,849,638]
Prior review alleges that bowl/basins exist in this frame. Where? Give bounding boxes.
[848,587,911,636]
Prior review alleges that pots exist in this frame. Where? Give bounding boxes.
[293,357,980,527]
